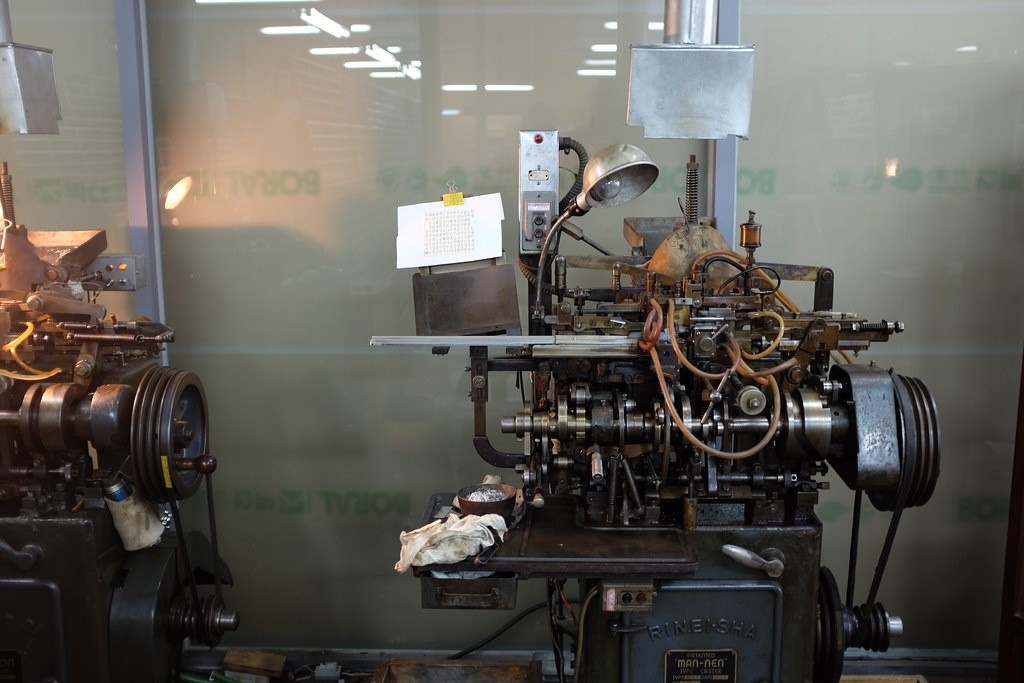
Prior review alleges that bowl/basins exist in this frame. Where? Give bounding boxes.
[458,484,517,518]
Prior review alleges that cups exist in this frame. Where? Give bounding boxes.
[104,479,130,502]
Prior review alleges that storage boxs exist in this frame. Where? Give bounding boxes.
[372,656,543,683]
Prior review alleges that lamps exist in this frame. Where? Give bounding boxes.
[535,142,660,308]
